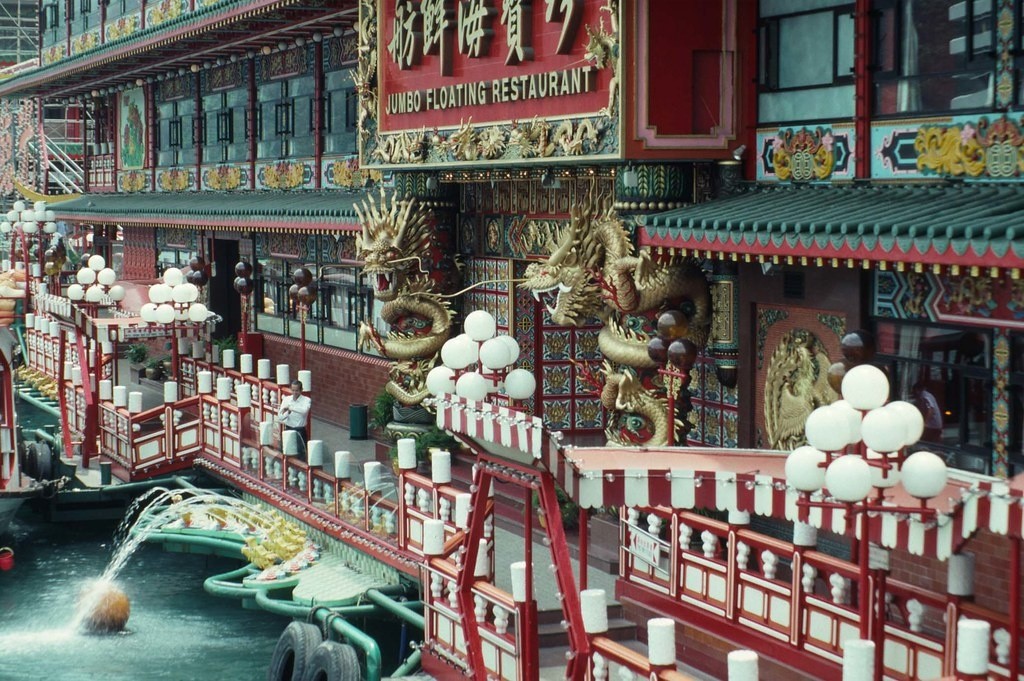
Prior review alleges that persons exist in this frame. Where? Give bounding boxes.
[16,239,74,282]
[911,381,944,443]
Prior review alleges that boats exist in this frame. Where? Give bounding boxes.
[0,0,1023,681]
